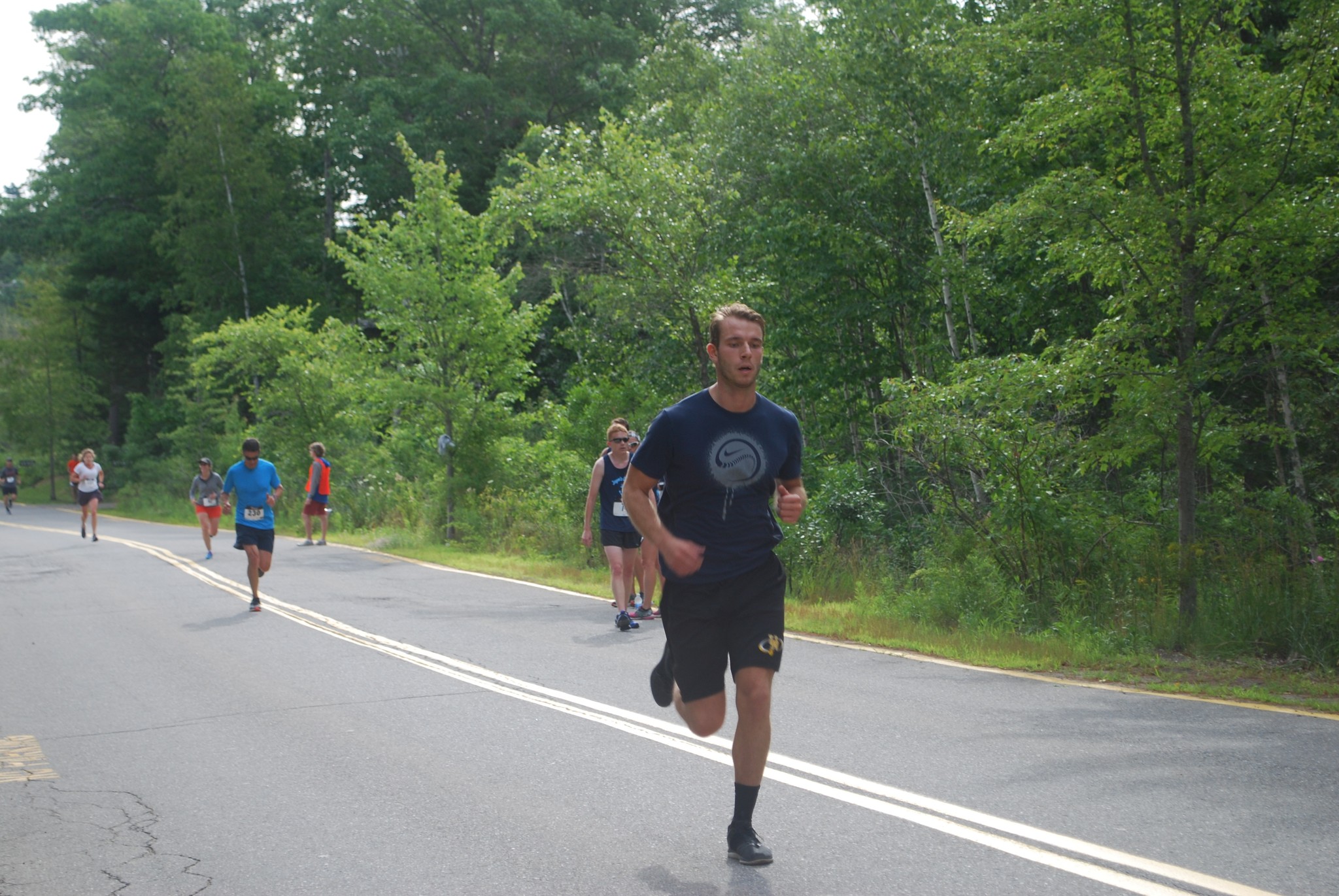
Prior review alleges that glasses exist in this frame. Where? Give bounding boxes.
[609,437,629,443]
[246,456,259,461]
[629,442,640,448]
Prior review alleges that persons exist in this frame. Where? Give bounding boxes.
[220,439,284,612]
[69,448,104,541]
[297,442,331,546]
[189,457,225,559]
[67,453,79,504]
[0,458,21,513]
[581,417,665,631]
[622,304,808,864]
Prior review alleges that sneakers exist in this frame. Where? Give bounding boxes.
[206,551,212,559]
[297,539,313,547]
[650,641,673,707]
[633,593,655,608]
[249,599,262,612]
[629,605,654,620]
[727,825,773,864]
[628,617,639,629]
[615,611,631,631]
[257,568,265,578]
[316,540,326,545]
[653,609,661,618]
[611,594,636,608]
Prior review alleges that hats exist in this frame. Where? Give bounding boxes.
[6,457,13,462]
[197,458,212,465]
